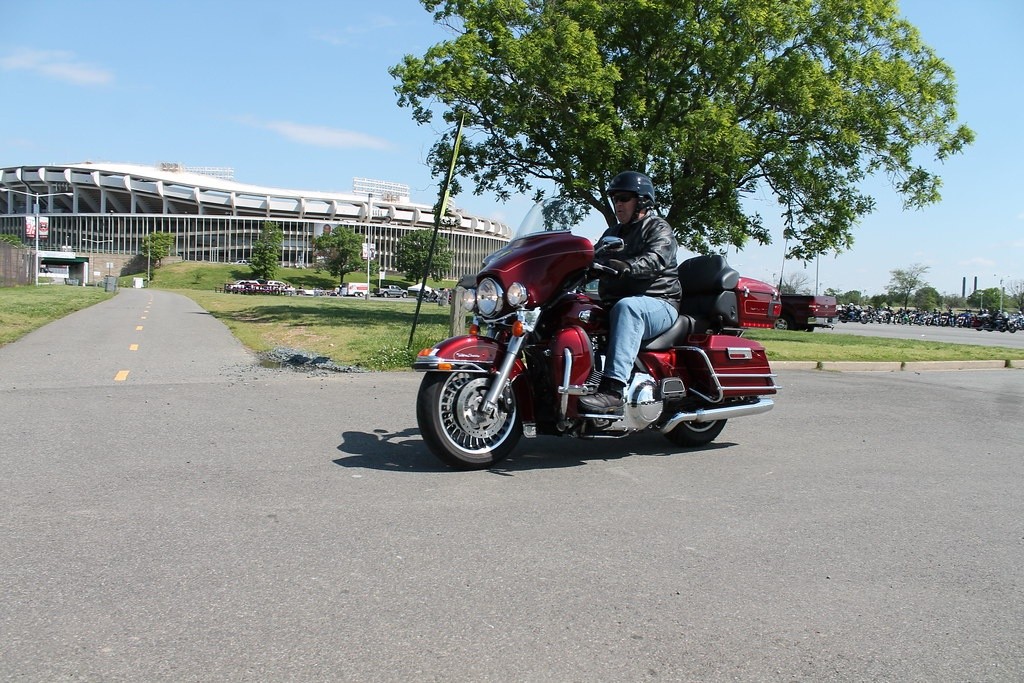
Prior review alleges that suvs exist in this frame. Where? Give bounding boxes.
[264,281,293,296]
[226,280,261,294]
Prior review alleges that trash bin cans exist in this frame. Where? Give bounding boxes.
[104,276,116,292]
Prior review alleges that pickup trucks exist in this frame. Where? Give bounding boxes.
[373,285,409,298]
[773,293,837,332]
[236,260,252,266]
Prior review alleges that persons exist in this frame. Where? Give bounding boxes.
[313,224,337,263]
[578,169,685,418]
[837,300,1023,334]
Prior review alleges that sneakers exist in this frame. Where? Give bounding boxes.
[577,390,624,415]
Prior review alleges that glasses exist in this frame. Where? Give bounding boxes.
[612,195,636,203]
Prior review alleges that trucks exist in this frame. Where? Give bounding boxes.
[334,283,369,298]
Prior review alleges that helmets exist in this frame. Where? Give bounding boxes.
[605,170,655,204]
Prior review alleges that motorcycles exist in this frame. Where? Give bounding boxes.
[835,303,1024,333]
[412,198,784,472]
[416,288,453,304]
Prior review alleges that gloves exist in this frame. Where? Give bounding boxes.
[603,259,631,279]
